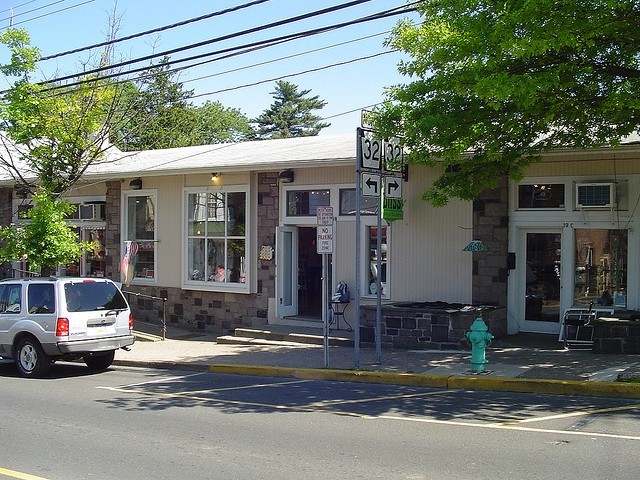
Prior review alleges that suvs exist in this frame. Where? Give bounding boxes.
[525,263,543,320]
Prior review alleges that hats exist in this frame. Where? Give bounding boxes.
[372,243,388,261]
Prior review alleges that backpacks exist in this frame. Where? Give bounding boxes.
[332,280,350,303]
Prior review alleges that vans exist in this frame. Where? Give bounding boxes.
[0,275,136,375]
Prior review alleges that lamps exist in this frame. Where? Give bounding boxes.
[211,172,218,181]
[129,178,142,190]
[279,171,294,184]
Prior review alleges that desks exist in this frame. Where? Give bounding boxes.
[327,301,353,332]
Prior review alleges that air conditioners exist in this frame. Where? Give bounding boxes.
[78,203,102,223]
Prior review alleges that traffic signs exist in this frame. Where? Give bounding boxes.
[316,205,334,255]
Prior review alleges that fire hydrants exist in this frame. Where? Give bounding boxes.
[466,316,495,373]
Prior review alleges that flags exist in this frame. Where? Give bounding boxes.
[121,241,140,288]
